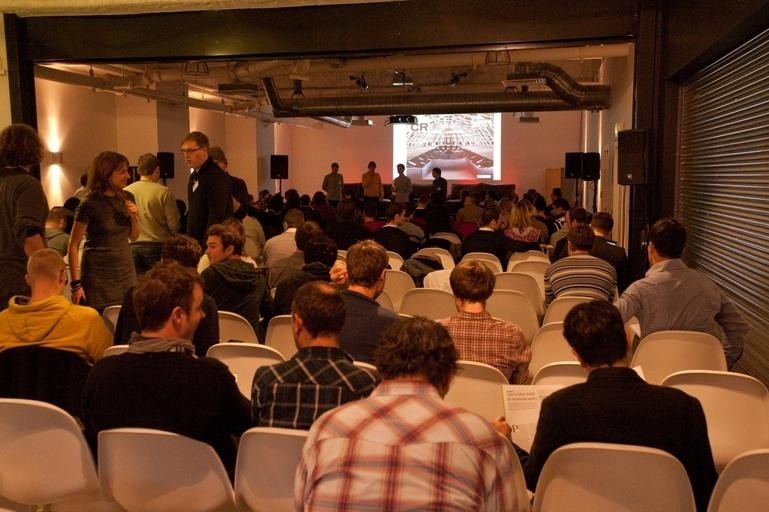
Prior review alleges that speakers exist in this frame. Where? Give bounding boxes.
[618,129,656,185]
[157,152,175,179]
[565,152,585,179]
[580,152,600,181]
[271,155,288,179]
[126,166,140,185]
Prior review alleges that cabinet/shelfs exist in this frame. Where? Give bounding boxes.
[545,167,577,210]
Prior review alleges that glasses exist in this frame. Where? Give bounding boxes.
[181,146,202,154]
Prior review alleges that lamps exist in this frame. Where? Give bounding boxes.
[392,70,414,87]
[449,72,468,87]
[356,79,369,90]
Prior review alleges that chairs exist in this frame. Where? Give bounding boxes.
[338,182,520,219]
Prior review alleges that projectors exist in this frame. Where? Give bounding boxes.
[389,115,418,125]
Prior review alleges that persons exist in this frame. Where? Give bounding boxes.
[610,217,749,373]
[0,122,625,365]
[492,295,720,512]
[291,314,533,511]
[435,259,533,387]
[77,262,252,492]
[247,280,379,432]
